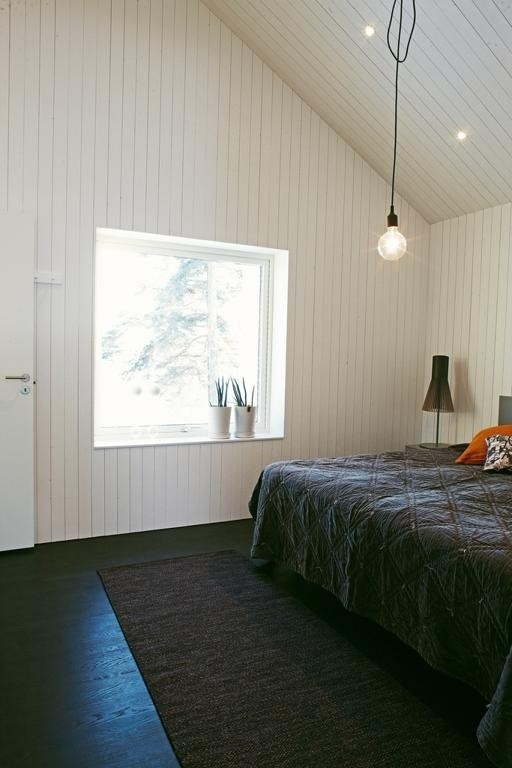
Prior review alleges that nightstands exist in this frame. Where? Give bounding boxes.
[405,445,430,450]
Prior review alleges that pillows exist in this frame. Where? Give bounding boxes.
[455,424,512,472]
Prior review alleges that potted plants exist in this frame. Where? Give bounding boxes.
[231,376,258,439]
[208,375,232,438]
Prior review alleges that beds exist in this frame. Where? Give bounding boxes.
[247,396,511,654]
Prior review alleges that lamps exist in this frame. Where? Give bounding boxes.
[377,0,416,262]
[420,354,455,449]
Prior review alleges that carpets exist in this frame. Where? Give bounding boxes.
[93,547,477,767]
